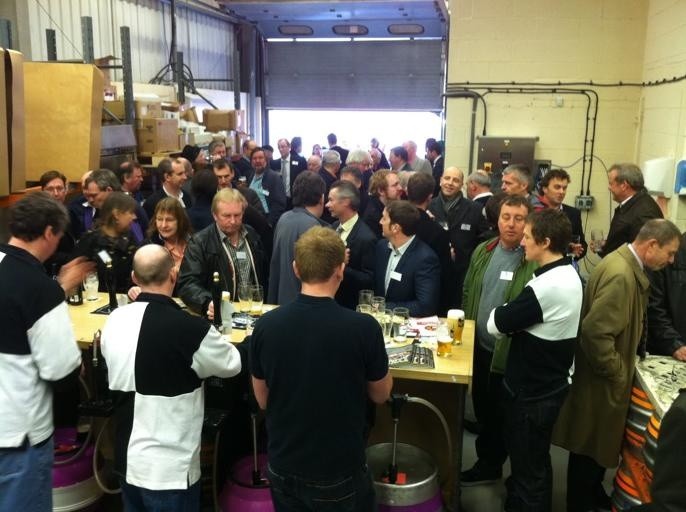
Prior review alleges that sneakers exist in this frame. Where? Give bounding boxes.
[462,464,501,487]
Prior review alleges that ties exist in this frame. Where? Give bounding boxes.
[280,161,288,182]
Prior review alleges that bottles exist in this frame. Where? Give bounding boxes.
[68,281,83,306]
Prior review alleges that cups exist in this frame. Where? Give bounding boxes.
[236,282,253,313]
[83,271,100,301]
[567,235,580,257]
[355,289,393,344]
[249,285,264,314]
[447,309,465,345]
[436,326,455,358]
[393,306,409,342]
[238,175,246,185]
[221,290,231,303]
[246,314,262,336]
[591,230,604,252]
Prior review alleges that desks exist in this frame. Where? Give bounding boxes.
[610,348,686,512]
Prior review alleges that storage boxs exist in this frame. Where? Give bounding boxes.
[0,49,252,196]
